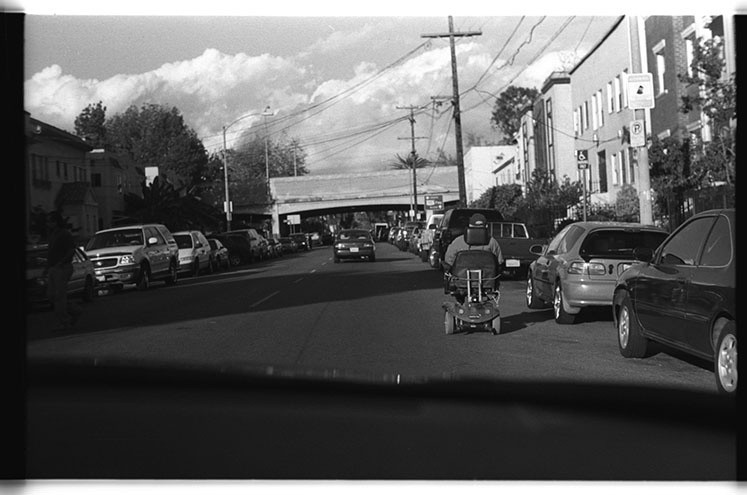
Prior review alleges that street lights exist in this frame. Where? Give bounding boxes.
[221,111,274,231]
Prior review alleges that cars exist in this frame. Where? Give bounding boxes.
[332,229,376,263]
[612,209,738,398]
[526,220,671,325]
[208,239,231,271]
[171,230,213,277]
[207,228,323,266]
[27,244,96,311]
[370,207,544,281]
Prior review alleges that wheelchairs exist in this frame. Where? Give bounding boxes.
[440,249,502,335]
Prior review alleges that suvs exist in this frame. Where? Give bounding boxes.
[84,223,180,297]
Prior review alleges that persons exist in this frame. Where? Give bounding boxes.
[444,213,504,276]
[38,212,76,333]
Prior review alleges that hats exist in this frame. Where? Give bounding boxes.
[468,213,488,226]
